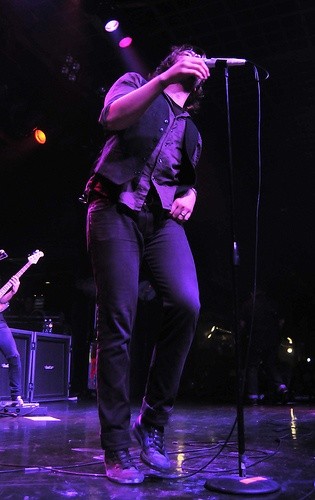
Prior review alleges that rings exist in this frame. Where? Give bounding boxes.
[180,211,186,217]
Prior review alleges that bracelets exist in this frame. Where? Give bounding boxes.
[192,188,197,196]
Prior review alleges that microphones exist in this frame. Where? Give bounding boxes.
[199,55,246,68]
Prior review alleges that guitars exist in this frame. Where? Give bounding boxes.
[0,249,45,313]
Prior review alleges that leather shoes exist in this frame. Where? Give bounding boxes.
[132,416,170,472]
[103,449,144,484]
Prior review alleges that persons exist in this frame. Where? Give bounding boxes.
[0,248,47,417]
[79,43,209,484]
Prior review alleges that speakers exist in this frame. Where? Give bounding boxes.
[0,328,73,403]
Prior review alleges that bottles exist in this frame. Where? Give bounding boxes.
[43,318,52,333]
